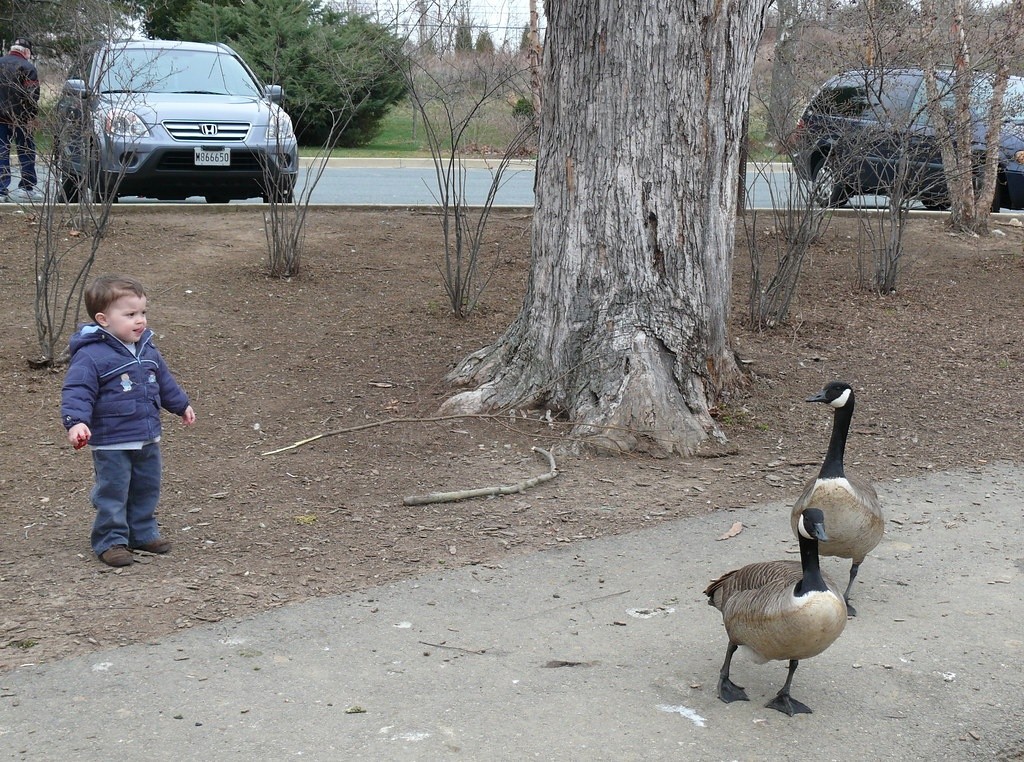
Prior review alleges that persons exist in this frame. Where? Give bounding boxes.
[0,37,40,203]
[61,276,196,565]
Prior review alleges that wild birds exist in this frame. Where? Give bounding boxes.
[790,381,883,615]
[701,508,849,717]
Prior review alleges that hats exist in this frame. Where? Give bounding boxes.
[14,39,34,55]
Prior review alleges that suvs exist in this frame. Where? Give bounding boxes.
[791,69,1023,213]
[56,40,298,204]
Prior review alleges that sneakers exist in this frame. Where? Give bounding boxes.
[133,538,171,553]
[97,544,134,566]
[19,190,42,200]
[0,195,10,203]
